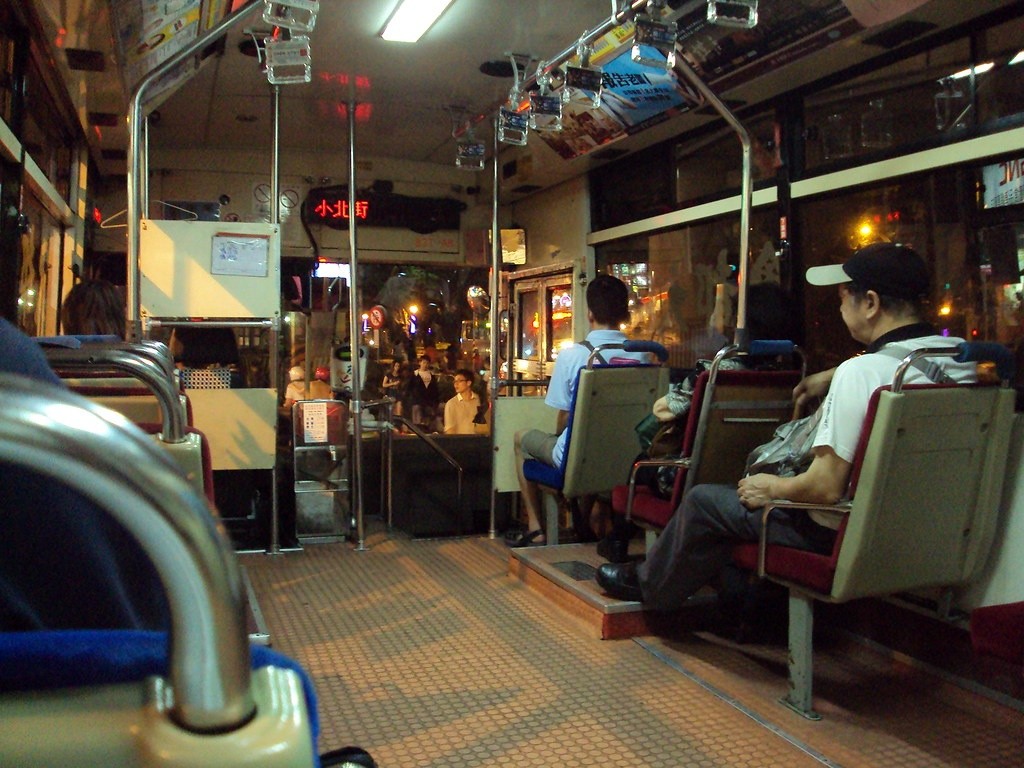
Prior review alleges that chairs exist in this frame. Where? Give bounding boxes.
[728,336,1019,726]
[518,333,673,544]
[610,328,816,587]
[0,339,323,768]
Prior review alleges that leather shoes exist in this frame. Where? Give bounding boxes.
[596,561,643,602]
[598,531,628,563]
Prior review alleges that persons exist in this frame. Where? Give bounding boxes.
[402,355,438,424]
[597,282,802,561]
[62,280,128,342]
[482,359,491,402]
[0,319,173,630]
[444,370,485,434]
[505,276,652,546]
[170,317,242,385]
[135,18,165,55]
[283,367,331,407]
[597,243,978,621]
[584,116,613,145]
[382,360,407,431]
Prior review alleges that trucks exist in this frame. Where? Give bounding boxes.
[459,318,533,363]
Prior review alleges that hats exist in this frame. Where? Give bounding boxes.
[804,242,934,301]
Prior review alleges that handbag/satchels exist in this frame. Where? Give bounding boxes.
[640,421,683,498]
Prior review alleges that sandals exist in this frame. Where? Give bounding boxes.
[572,526,596,544]
[503,527,547,548]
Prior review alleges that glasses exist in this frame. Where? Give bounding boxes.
[4,210,30,247]
[452,379,468,384]
